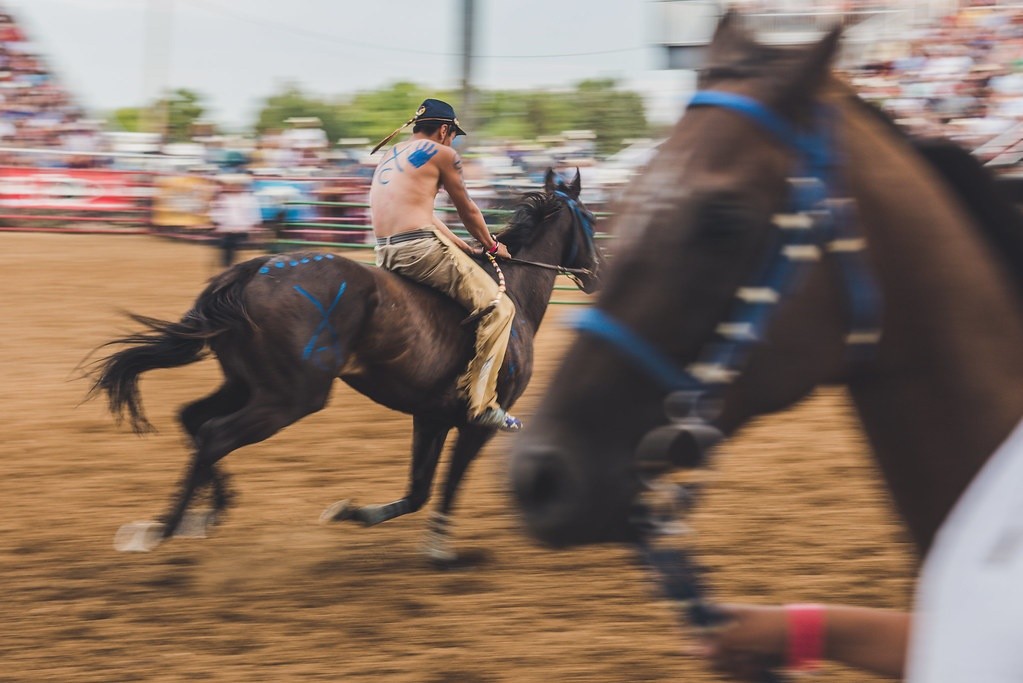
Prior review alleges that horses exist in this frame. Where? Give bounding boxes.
[65,166,604,569]
[504,5,1022,565]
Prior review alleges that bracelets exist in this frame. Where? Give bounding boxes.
[786,604,825,661]
[489,240,498,254]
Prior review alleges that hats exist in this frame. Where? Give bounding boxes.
[414,98,467,136]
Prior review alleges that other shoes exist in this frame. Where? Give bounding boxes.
[466,408,523,432]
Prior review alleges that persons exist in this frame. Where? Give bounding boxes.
[371,99,524,432]
[207,173,261,268]
[691,418,1023,683]
